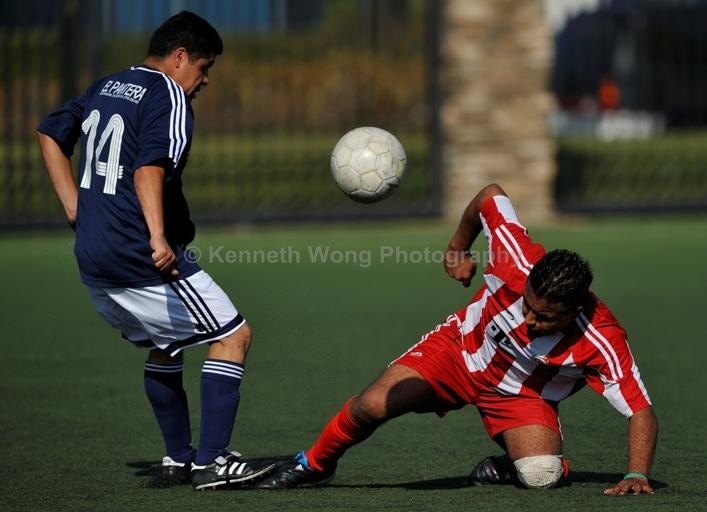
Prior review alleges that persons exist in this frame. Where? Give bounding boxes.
[36,9,276,492]
[262,183,659,498]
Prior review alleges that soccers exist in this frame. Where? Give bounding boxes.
[332,127,407,204]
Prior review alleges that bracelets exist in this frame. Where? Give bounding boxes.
[622,472,648,480]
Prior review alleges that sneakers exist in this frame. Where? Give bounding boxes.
[469,456,513,486]
[190,450,278,492]
[240,449,336,489]
[160,448,197,485]
